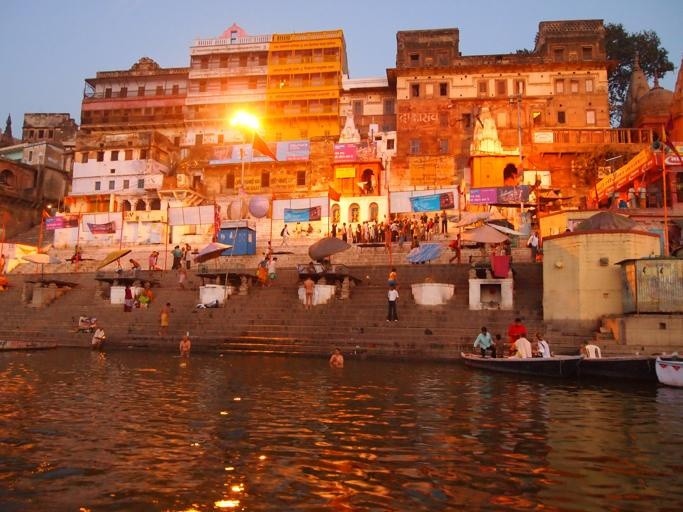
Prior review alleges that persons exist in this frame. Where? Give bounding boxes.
[180,336,191,358]
[473,318,601,360]
[329,348,344,369]
[386,285,399,323]
[257,222,313,289]
[92,327,106,349]
[387,268,398,289]
[71,246,81,264]
[124,284,133,312]
[332,210,461,265]
[0,275,8,291]
[527,232,540,264]
[130,259,141,271]
[79,315,88,327]
[158,302,170,336]
[1,254,7,274]
[149,251,159,272]
[629,186,636,208]
[304,276,315,310]
[170,244,191,270]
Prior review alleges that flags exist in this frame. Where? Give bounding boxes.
[328,186,341,202]
[253,134,280,164]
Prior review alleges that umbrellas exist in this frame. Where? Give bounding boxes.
[460,225,508,244]
[21,254,62,280]
[194,243,232,268]
[97,250,132,271]
[455,212,493,229]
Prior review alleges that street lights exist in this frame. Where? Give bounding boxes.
[228,109,261,197]
[505,91,526,217]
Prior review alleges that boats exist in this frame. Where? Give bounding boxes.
[650,352,683,387]
[549,350,656,383]
[458,349,587,385]
